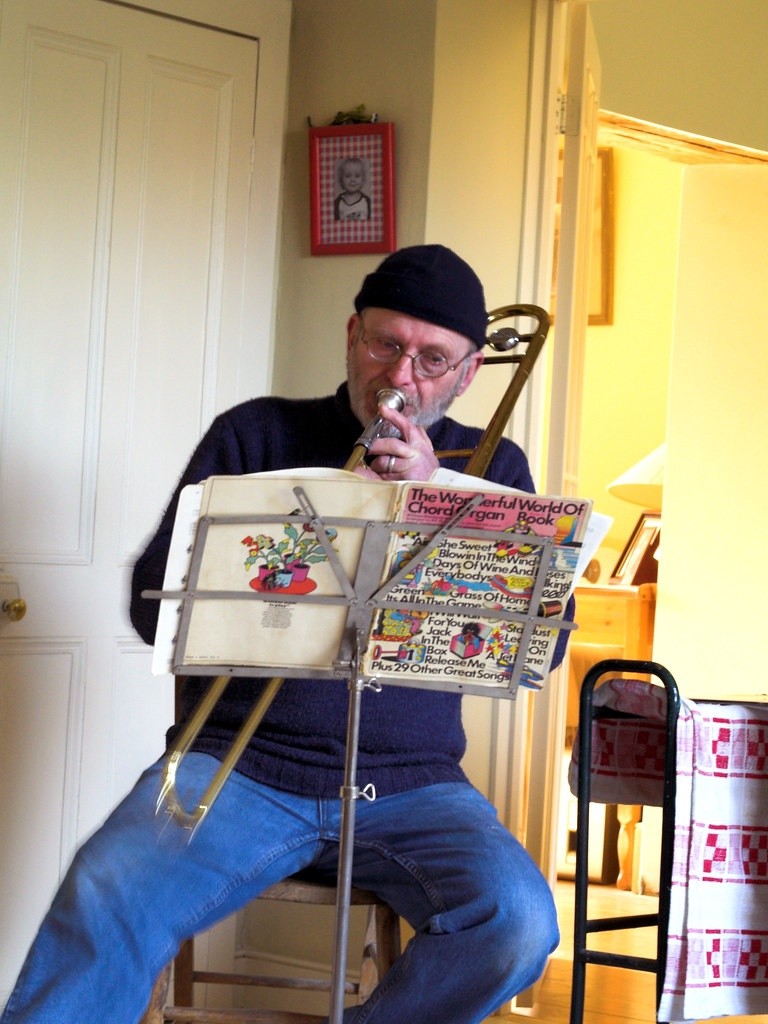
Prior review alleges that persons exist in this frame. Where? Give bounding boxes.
[0,242,561,1024]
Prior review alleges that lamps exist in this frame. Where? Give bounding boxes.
[609,449,668,506]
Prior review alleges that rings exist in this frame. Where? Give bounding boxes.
[385,455,396,474]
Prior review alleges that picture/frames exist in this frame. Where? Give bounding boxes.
[610,512,662,580]
[546,145,618,328]
[308,121,397,257]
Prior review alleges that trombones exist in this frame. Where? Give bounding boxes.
[161,302,553,829]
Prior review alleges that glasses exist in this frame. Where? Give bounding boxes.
[361,331,476,378]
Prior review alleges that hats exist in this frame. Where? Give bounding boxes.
[354,244,487,345]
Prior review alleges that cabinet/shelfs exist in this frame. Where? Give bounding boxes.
[571,660,768,1024]
[556,583,660,886]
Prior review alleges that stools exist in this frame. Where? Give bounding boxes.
[162,877,401,1024]
